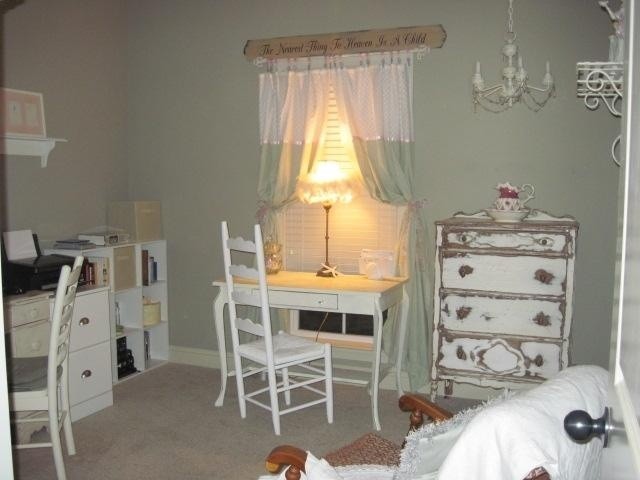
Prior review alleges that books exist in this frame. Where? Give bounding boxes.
[153,261,158,281]
[141,249,149,287]
[53,236,98,285]
[149,257,154,283]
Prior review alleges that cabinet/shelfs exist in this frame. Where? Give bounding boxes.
[9,238,171,445]
[429,208,580,405]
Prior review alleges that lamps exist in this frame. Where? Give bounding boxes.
[295,160,355,278]
[470,0,555,110]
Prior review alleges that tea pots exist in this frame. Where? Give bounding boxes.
[494,182,536,211]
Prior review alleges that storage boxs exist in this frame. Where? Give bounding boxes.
[114,245,137,291]
[106,199,162,243]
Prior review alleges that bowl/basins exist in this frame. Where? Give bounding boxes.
[483,207,531,223]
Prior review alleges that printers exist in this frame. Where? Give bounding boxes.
[0,234,89,292]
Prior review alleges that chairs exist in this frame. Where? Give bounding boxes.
[7,254,86,480]
[220,219,335,437]
[264,362,612,479]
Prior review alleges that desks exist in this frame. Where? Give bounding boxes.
[211,270,411,432]
